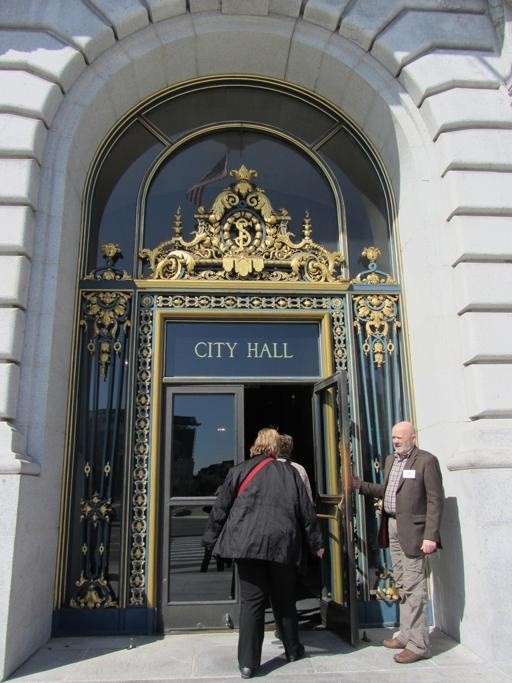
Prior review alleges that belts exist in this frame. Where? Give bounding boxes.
[385,513,397,520]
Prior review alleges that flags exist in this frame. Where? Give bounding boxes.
[186,150,230,207]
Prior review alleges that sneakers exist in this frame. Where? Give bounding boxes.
[394,648,426,664]
[285,644,305,663]
[382,636,407,650]
[239,665,259,679]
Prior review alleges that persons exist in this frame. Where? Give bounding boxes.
[351,422,443,663]
[201,428,329,679]
